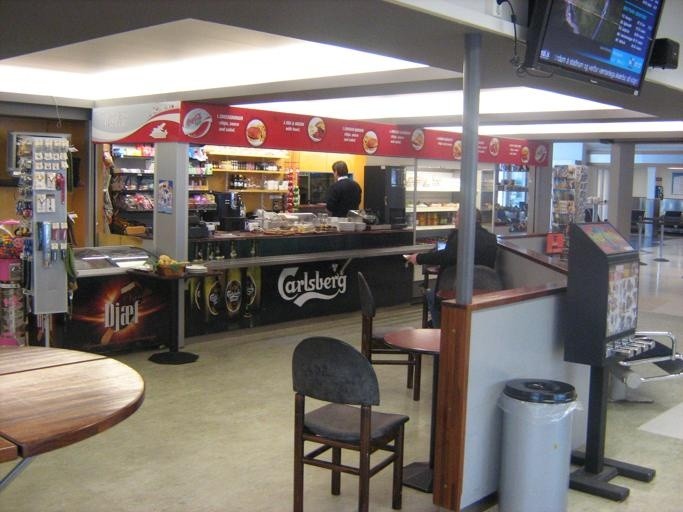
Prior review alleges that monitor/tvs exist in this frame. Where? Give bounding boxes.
[6,131,72,180]
[522,1,665,97]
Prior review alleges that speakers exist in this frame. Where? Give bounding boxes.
[648,37,679,71]
[72,157,81,186]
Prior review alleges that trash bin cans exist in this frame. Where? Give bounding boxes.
[499,379,577,512]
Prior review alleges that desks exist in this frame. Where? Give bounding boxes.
[0,345,146,491]
[434,289,496,300]
[126,266,224,365]
[383,327,442,493]
[422,263,440,328]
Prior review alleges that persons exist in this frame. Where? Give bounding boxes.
[404,206,497,329]
[323,161,362,217]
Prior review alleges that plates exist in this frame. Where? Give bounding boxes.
[327,216,366,233]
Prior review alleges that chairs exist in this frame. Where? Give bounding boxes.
[293,336,409,512]
[431,262,504,331]
[357,271,421,401]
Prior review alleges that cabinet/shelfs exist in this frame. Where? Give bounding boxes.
[98,141,291,238]
[553,165,588,234]
[405,207,458,281]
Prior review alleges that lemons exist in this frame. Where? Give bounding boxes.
[157,254,177,265]
